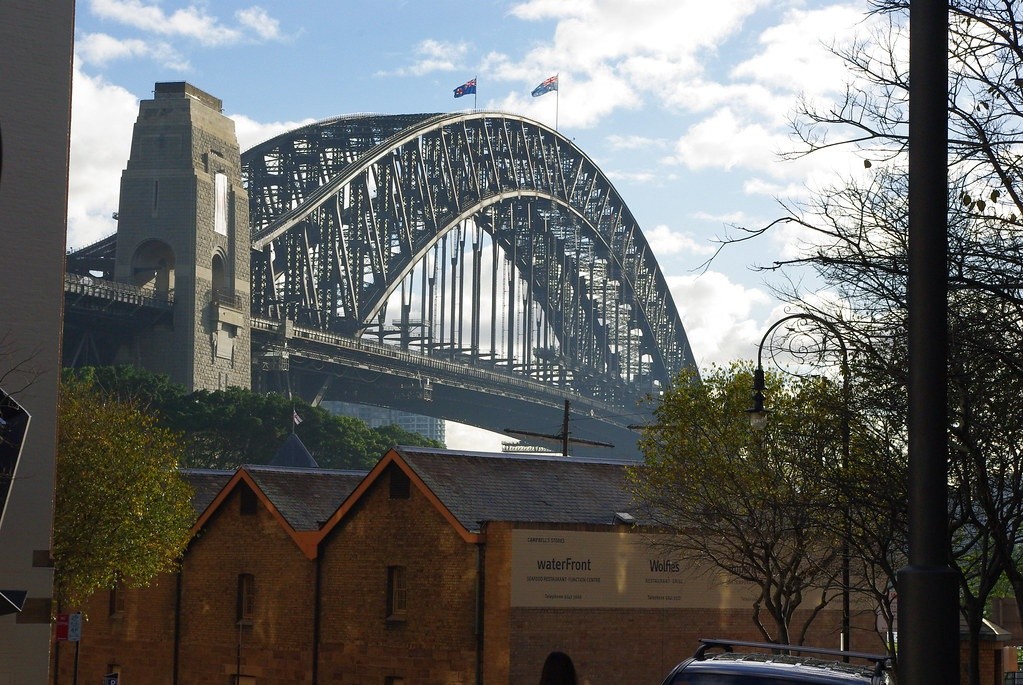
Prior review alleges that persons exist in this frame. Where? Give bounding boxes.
[539,652,577,685]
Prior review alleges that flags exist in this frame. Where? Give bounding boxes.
[453,79,475,98]
[531,75,558,97]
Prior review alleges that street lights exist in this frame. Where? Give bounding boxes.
[744,314,851,664]
